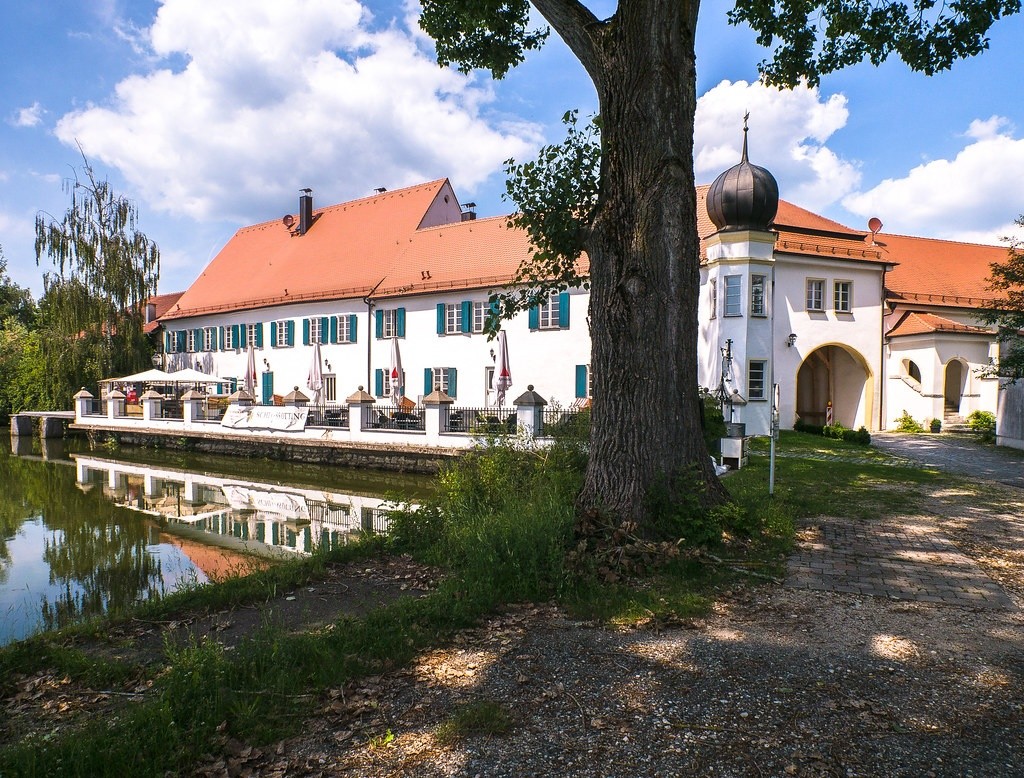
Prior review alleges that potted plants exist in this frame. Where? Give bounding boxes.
[930,418,941,433]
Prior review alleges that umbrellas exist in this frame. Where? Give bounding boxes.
[111,367,236,418]
[114,493,235,525]
[306,342,326,426]
[492,328,513,408]
[243,343,258,406]
[388,336,405,430]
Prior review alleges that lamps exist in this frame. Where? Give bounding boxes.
[788,334,798,347]
[325,358,330,370]
[490,348,496,361]
[264,359,269,367]
[196,362,202,370]
